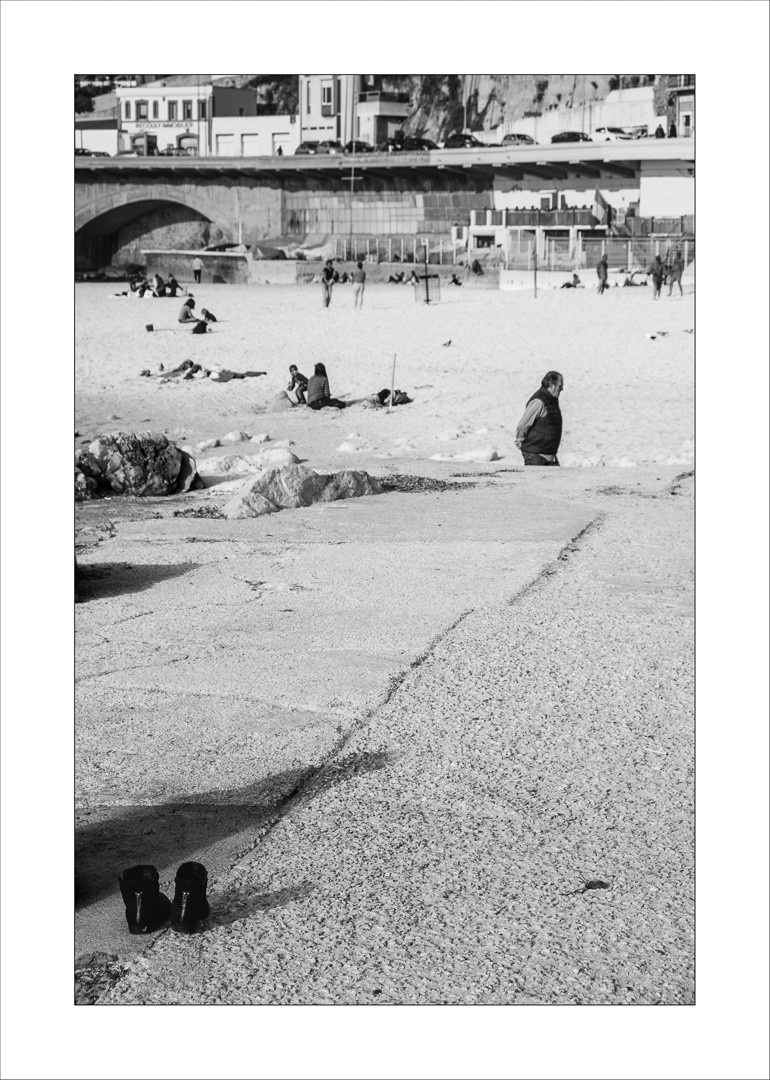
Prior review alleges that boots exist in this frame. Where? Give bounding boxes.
[169,861,209,932]
[118,865,171,934]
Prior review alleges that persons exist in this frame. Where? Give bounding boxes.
[192,255,204,283]
[516,370,564,465]
[307,362,346,410]
[130,273,200,323]
[277,145,283,156]
[321,250,685,310]
[655,124,665,138]
[669,120,677,137]
[286,365,308,404]
